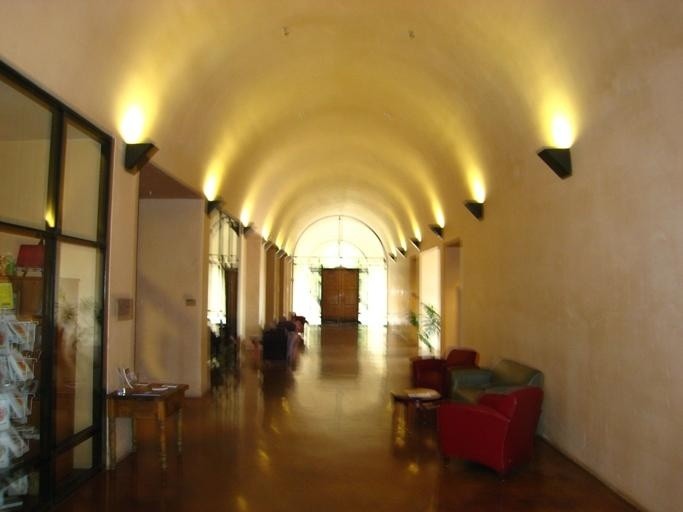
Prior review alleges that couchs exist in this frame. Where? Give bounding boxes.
[437,387,544,474]
[450,358,544,404]
[412,348,480,394]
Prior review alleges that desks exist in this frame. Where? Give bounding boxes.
[106,381,190,469]
